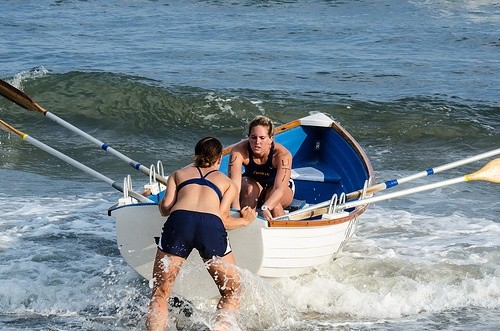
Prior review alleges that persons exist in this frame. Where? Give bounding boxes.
[146,136,257,331]
[227,116,294,218]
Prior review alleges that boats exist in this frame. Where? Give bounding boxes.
[107,108,377,304]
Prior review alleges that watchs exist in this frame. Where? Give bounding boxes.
[261,206,270,211]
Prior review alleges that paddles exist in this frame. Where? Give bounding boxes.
[0,118,155,204]
[0,78,168,186]
[269,157,500,224]
[269,148,500,219]
